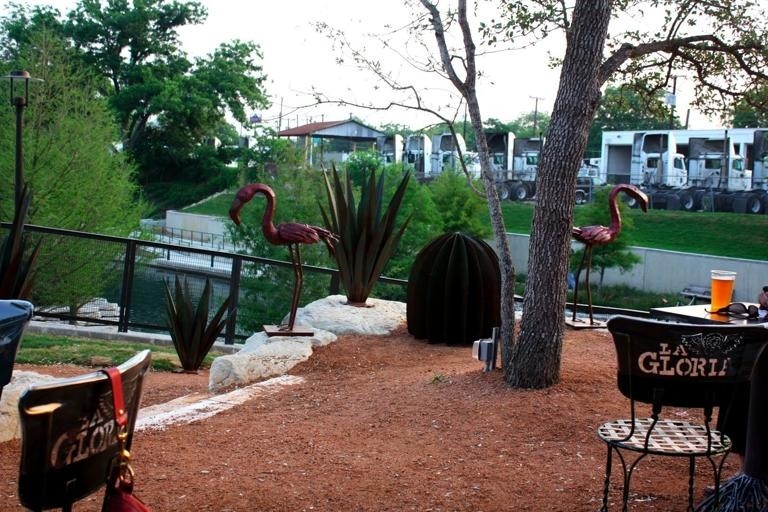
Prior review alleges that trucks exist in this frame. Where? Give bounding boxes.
[628,127,768,216]
[378,130,607,206]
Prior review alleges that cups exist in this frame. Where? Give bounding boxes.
[711,269,736,322]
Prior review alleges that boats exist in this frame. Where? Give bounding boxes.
[30,294,133,330]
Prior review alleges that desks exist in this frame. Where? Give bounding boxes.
[651,300,767,510]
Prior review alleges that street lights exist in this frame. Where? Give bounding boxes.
[0,68,45,227]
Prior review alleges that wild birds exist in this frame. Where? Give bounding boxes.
[570,183,649,326]
[228,183,343,332]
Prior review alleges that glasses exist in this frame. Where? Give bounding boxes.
[704,302,760,319]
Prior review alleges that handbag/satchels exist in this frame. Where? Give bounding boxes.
[104,478,152,512]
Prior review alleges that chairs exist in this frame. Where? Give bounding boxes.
[15,348,152,512]
[592,313,764,512]
